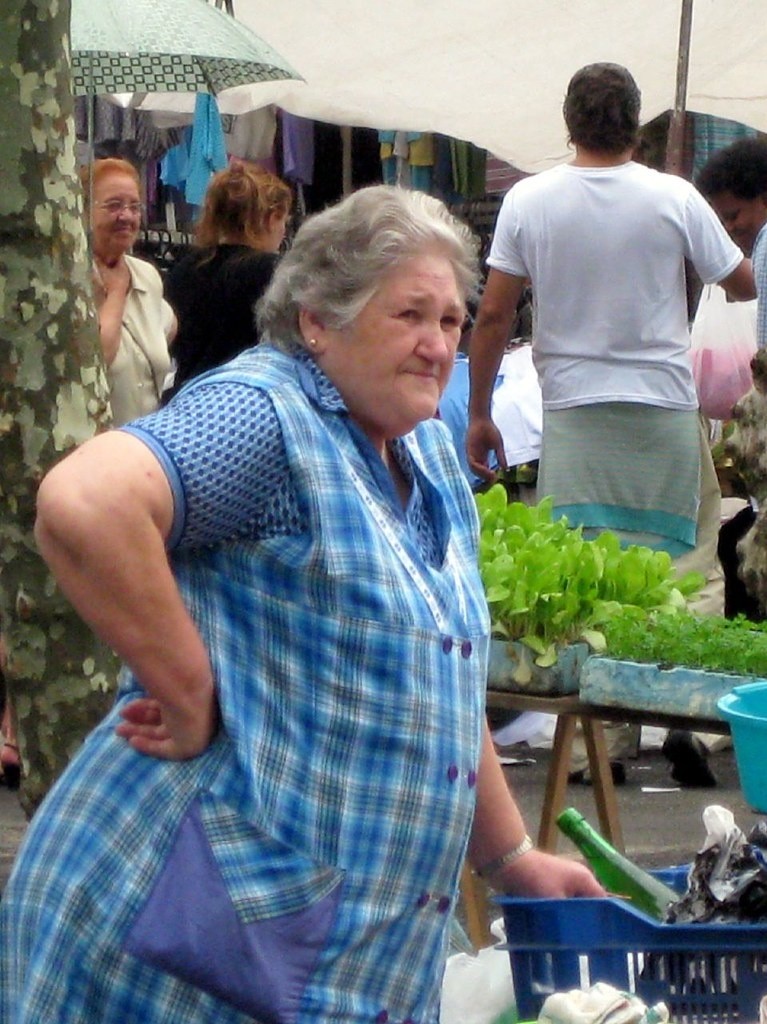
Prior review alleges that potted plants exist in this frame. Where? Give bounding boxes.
[469,484,705,696]
[578,607,767,720]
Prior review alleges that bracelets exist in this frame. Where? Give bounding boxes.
[470,835,533,879]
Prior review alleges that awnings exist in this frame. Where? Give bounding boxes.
[100,0,767,174]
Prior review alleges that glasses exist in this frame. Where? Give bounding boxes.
[93,200,146,215]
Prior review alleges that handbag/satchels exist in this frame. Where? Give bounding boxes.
[689,283,759,419]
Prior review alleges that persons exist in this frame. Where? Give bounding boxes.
[0,185,608,1024]
[467,62,758,785]
[0,137,767,789]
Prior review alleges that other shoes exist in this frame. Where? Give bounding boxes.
[662,729,715,787]
[568,760,626,785]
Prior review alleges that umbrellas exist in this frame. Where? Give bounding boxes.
[70,0,309,265]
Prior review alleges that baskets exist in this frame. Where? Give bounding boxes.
[495,866,767,1024]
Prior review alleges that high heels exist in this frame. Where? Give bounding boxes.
[1,743,22,790]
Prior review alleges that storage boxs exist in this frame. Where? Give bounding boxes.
[487,864,767,1024]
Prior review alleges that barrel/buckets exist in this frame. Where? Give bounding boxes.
[715,681,767,814]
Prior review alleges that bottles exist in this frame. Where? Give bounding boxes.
[555,807,681,921]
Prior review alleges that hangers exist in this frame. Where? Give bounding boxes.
[132,226,196,271]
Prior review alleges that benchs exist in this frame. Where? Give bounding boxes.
[460,688,731,951]
